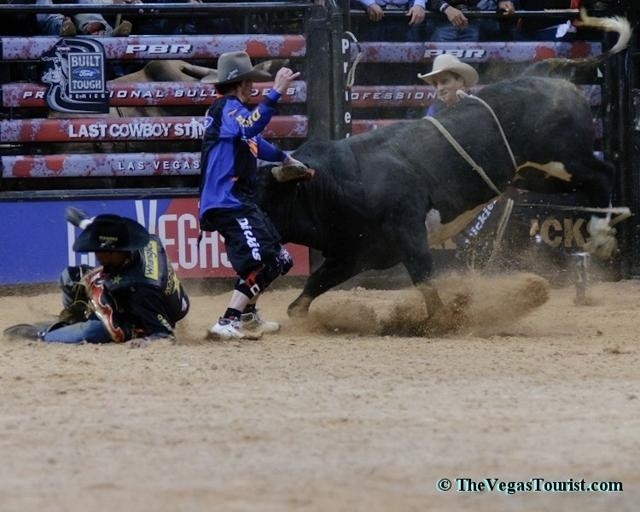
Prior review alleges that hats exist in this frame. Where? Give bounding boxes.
[73,214,150,253]
[417,53,479,87]
[201,50,289,85]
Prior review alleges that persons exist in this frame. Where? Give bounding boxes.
[197,50,317,342]
[414,51,514,277]
[3,204,190,344]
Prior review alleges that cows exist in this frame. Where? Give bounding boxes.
[237,6,637,331]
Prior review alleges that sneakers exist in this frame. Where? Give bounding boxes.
[210,311,281,340]
[3,320,54,339]
